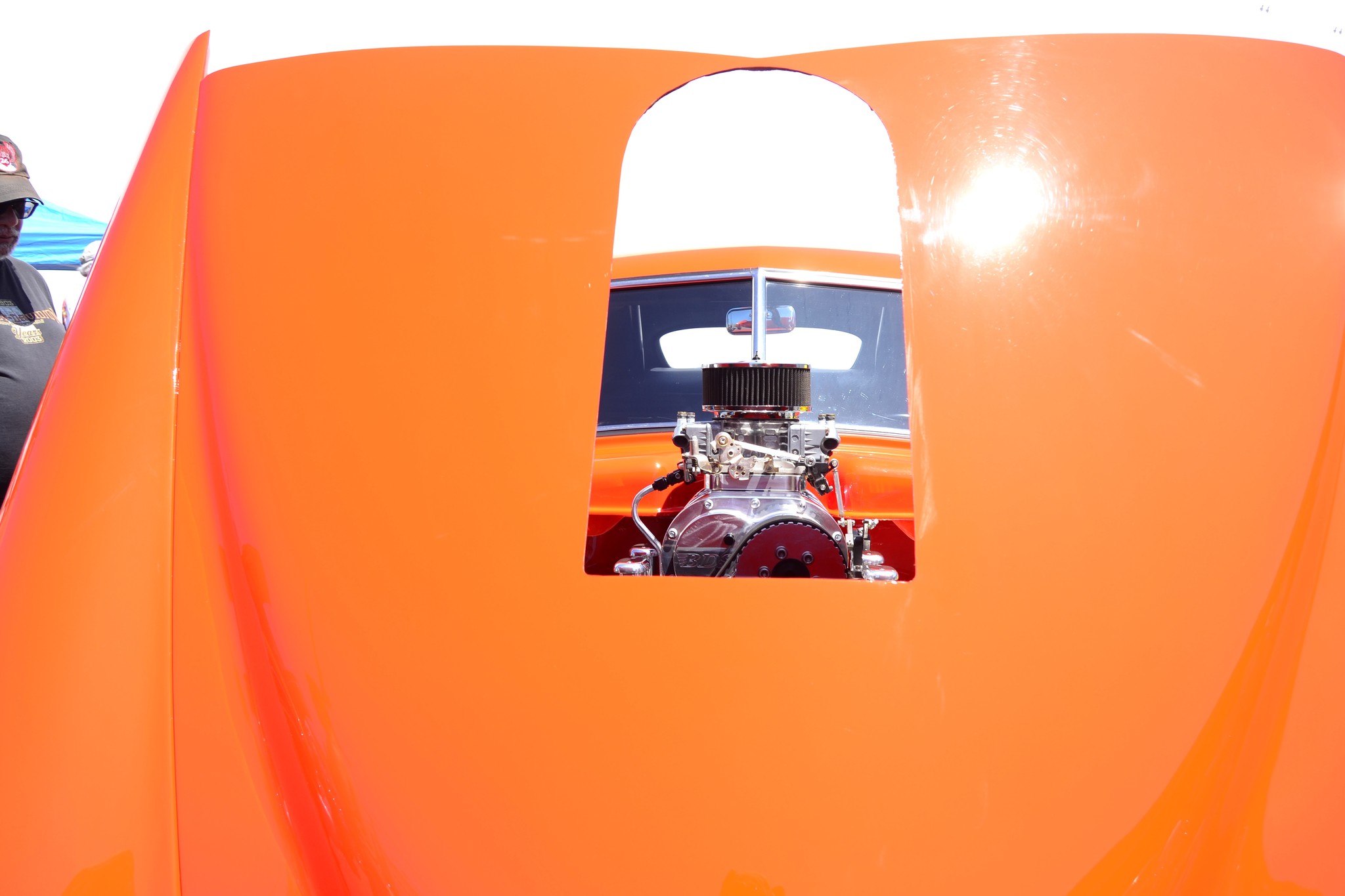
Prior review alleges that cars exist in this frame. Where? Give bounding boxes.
[0,33,1345,894]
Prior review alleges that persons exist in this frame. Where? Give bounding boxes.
[0,138,68,499]
[79,240,104,278]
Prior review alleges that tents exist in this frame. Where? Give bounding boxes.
[8,197,109,271]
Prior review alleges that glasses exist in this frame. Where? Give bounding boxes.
[0,198,39,219]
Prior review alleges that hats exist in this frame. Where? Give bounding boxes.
[0,134,44,206]
